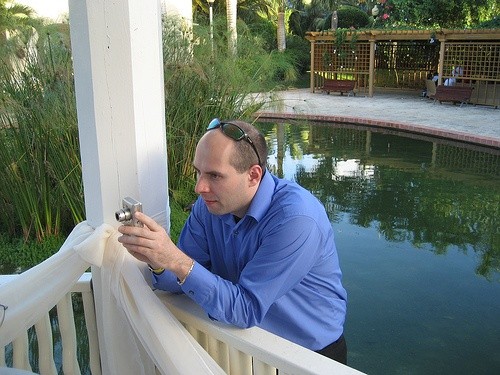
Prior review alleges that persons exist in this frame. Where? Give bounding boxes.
[118,118,347,365]
[428,64,464,86]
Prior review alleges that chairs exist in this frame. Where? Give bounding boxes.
[424,78,437,100]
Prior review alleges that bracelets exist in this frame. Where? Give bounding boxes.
[148,265,164,273]
[177,259,194,285]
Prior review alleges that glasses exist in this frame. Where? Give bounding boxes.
[207,118,261,167]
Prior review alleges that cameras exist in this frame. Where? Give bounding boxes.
[116,196,144,228]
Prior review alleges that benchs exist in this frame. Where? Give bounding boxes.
[428,84,475,110]
[320,79,357,97]
